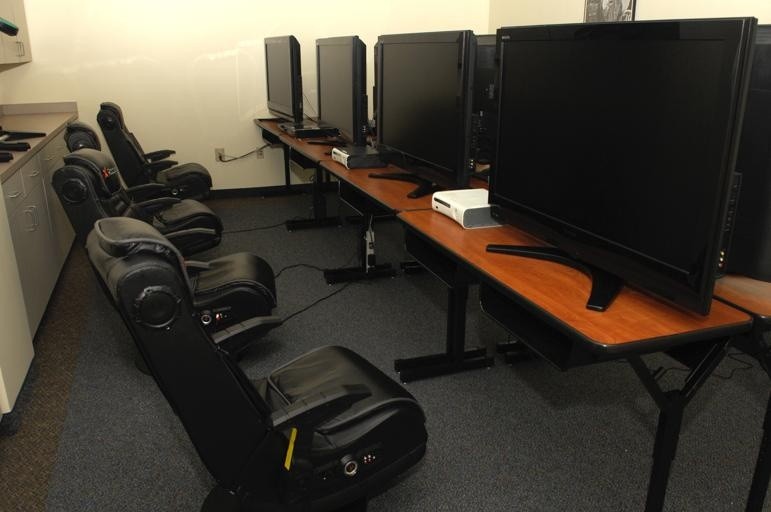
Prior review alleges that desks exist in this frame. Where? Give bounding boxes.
[319,157,490,283]
[394,209,771,511]
[254,118,397,228]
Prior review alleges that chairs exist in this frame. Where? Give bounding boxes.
[85,217,428,511]
[63,123,224,258]
[51,148,276,375]
[97,102,212,204]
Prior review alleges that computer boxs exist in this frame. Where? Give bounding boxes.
[330,148,388,171]
[431,187,505,229]
[279,121,341,139]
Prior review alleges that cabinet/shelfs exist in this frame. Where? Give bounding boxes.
[5,127,78,338]
[1,0,33,70]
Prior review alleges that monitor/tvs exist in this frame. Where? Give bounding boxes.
[313,36,369,149]
[367,32,499,203]
[724,23,771,287]
[263,35,305,127]
[486,17,759,317]
